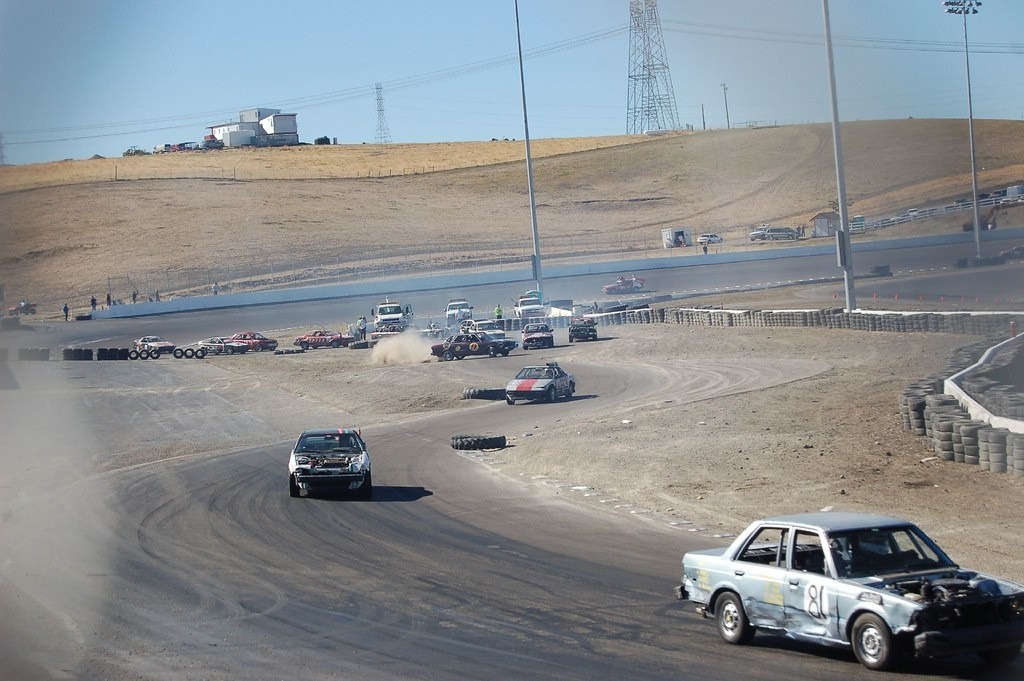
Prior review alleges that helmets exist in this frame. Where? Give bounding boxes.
[545,368,554,375]
[858,530,890,555]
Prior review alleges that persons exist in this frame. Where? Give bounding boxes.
[357,315,367,340]
[797,225,805,237]
[592,302,598,310]
[703,244,707,254]
[678,234,683,247]
[20,283,219,322]
[495,304,502,319]
[455,307,465,323]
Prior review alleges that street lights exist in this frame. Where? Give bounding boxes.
[943,0,984,261]
[720,83,731,131]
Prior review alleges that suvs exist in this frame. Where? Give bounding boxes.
[444,302,475,326]
[749,227,768,241]
[764,227,803,241]
[567,318,598,344]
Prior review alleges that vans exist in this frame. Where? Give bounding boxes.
[8,303,37,316]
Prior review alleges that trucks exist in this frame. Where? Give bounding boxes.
[371,302,413,330]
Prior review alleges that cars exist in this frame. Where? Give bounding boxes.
[133,336,177,353]
[430,333,518,361]
[674,511,1024,676]
[286,428,373,501]
[696,233,721,244]
[198,336,249,355]
[506,362,576,406]
[460,319,507,341]
[521,323,556,350]
[602,278,646,296]
[230,332,279,352]
[512,297,552,319]
[908,209,918,216]
[123,141,225,156]
[293,329,354,350]
